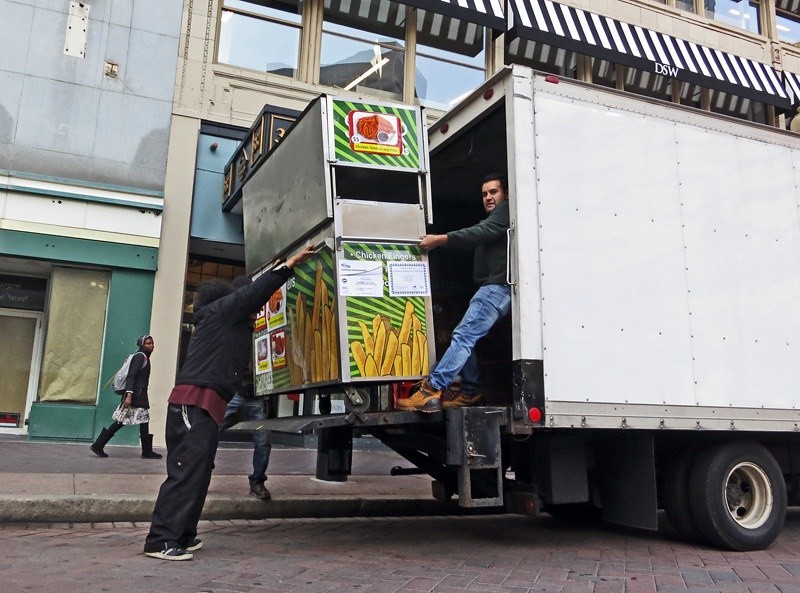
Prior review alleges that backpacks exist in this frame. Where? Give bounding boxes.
[114,352,147,395]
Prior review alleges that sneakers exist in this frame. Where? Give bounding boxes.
[182,539,202,551]
[442,393,484,410]
[396,387,442,412]
[146,546,193,560]
[250,484,271,499]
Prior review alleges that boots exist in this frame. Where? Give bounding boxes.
[91,427,115,457]
[139,434,163,459]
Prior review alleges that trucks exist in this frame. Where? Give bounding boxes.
[224,57,800,553]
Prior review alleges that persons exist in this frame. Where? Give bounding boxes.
[394,174,511,413]
[141,241,315,564]
[90,335,163,460]
[222,354,277,501]
